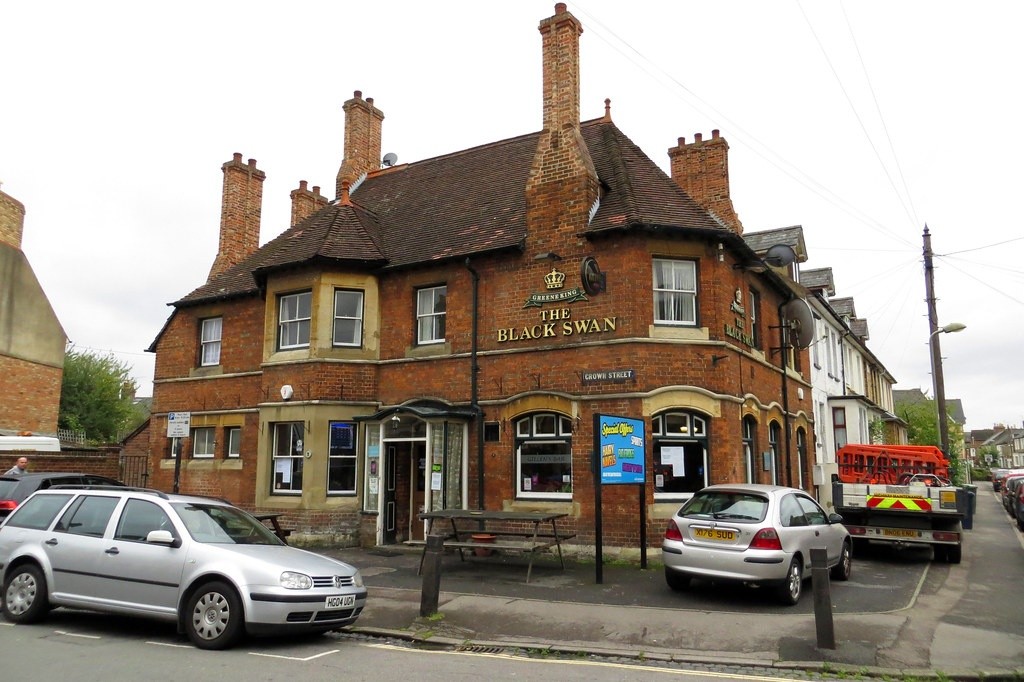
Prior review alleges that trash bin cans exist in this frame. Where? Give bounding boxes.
[961,484,978,514]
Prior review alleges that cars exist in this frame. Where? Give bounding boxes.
[990,468,1024,531]
[662,482,853,605]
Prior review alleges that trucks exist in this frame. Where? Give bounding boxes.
[831,441,964,564]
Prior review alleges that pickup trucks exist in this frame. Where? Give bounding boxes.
[1,484,369,650]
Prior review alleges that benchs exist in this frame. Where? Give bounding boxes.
[227,526,296,541]
[402,528,576,550]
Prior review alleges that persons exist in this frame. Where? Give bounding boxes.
[4,457,28,475]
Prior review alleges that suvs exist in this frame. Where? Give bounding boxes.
[0,470,125,536]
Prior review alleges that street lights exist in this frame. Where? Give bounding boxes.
[929,322,966,450]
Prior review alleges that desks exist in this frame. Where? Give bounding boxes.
[211,509,288,544]
[417,509,569,576]
